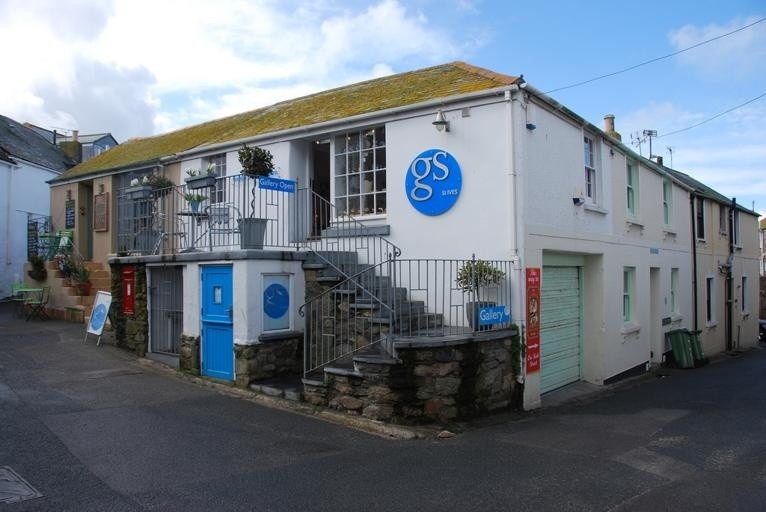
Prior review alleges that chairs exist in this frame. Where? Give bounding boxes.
[151,212,187,255]
[205,203,243,251]
[9,283,50,322]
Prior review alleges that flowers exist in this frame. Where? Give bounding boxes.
[129,176,149,187]
[184,163,217,176]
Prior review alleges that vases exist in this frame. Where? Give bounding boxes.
[184,175,215,189]
[125,186,152,200]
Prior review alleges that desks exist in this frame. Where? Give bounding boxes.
[177,212,209,252]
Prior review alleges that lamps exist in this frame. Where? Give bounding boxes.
[432,109,449,133]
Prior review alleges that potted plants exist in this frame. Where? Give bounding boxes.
[454,260,506,331]
[53,246,92,296]
[183,193,208,212]
[28,254,47,281]
[236,143,276,250]
[137,175,176,256]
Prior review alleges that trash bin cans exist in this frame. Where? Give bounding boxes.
[237,218,268,249]
[689,330,704,361]
[665,329,696,369]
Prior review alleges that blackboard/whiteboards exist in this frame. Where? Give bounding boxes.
[28,223,38,261]
[65,200,75,228]
[87,291,113,335]
